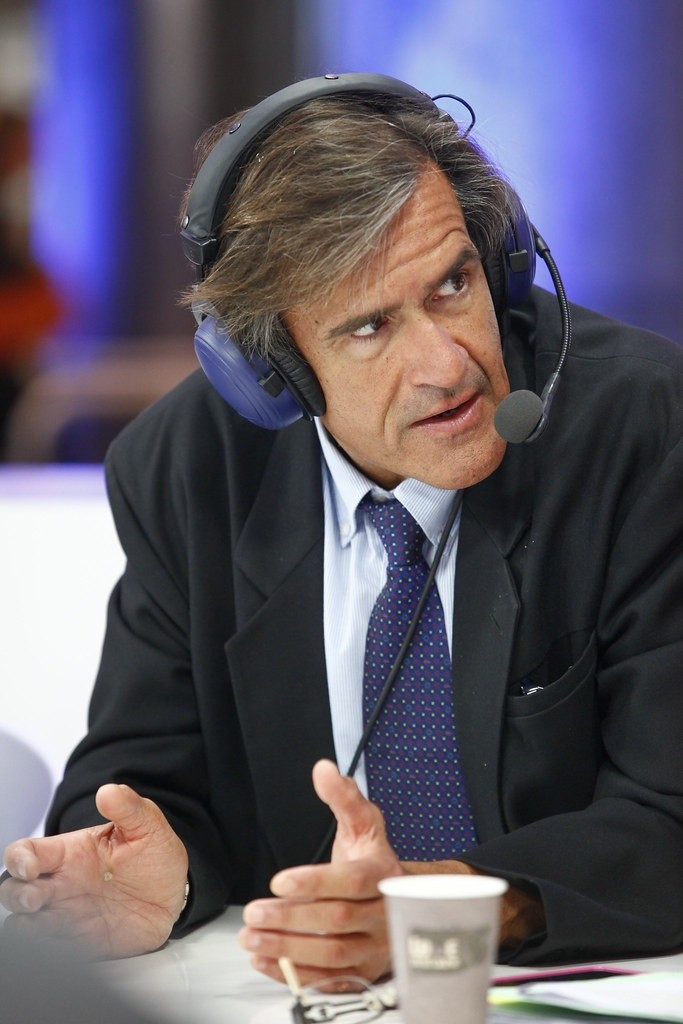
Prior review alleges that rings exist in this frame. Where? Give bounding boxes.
[338,981,349,992]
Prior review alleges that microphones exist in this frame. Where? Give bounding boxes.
[494,232,569,442]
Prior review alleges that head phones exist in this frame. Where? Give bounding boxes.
[181,70,542,429]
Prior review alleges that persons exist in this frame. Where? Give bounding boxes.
[1,90,683,996]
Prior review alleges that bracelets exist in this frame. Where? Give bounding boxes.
[181,878,189,910]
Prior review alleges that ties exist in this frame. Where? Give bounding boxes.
[358,494,477,861]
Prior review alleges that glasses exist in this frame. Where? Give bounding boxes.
[279,956,397,1024]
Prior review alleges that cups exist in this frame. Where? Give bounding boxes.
[378,874,508,1024]
